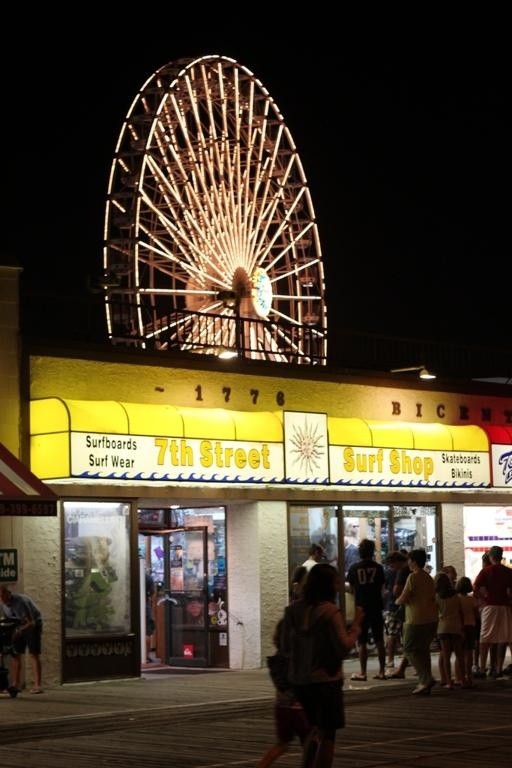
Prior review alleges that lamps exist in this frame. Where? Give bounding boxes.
[184,344,238,359]
[335,505,390,511]
[391,364,436,379]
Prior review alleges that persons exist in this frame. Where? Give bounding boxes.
[303,542,325,573]
[343,517,361,546]
[170,544,182,567]
[253,562,364,768]
[347,537,512,694]
[310,507,336,561]
[0,585,44,700]
[290,566,307,603]
[144,573,157,661]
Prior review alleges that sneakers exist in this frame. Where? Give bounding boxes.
[350,661,512,694]
